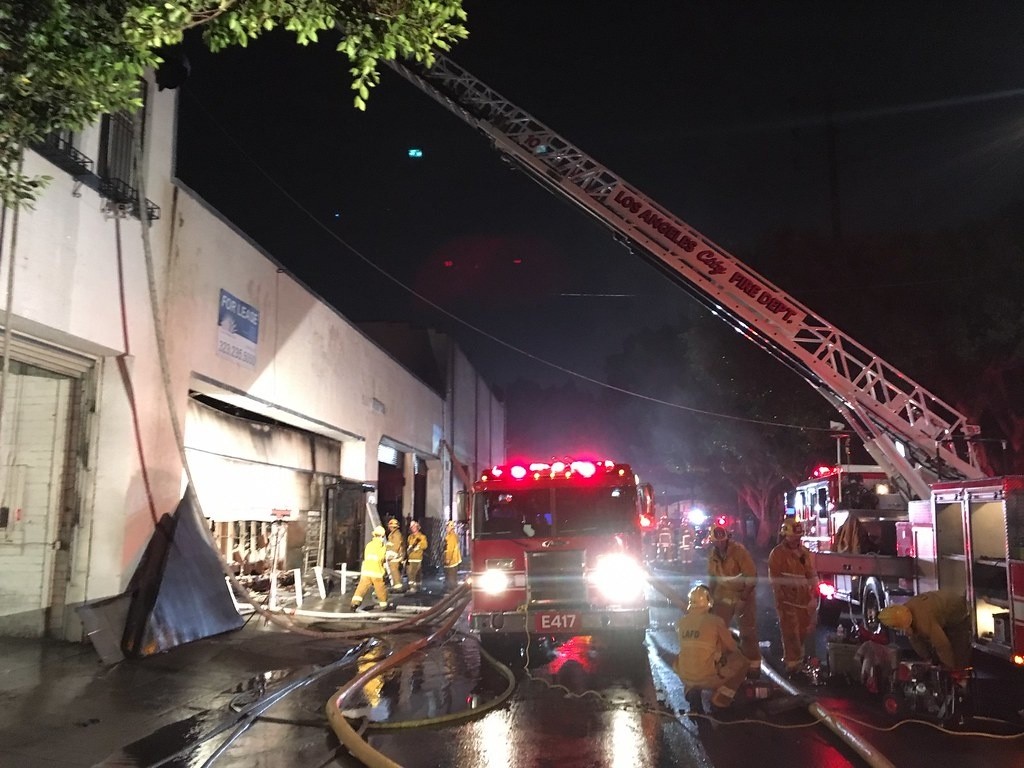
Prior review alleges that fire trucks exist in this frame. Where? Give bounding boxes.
[437,439,656,655]
[364,25,1024,731]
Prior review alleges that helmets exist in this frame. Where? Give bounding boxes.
[372,526,385,536]
[408,519,421,529]
[445,520,455,529]
[709,524,730,543]
[877,602,912,629]
[387,519,400,528]
[780,518,803,536]
[686,585,715,611]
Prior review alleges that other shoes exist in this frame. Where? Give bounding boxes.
[351,605,358,611]
[708,702,732,721]
[689,700,704,714]
[382,602,398,611]
[389,587,405,594]
[746,670,761,680]
[404,591,420,598]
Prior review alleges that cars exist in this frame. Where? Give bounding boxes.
[650,506,737,569]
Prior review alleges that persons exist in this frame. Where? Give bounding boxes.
[349,518,462,612]
[707,527,762,678]
[671,585,751,715]
[767,519,821,672]
[877,589,973,708]
